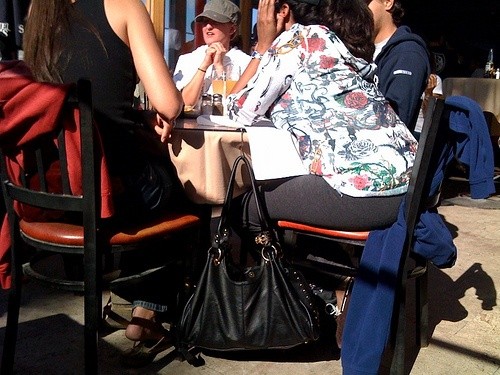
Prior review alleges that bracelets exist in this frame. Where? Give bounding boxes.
[197,68,206,73]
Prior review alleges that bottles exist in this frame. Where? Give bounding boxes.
[201,93,212,115]
[484,49,495,79]
[212,92,224,116]
[496,68,499,79]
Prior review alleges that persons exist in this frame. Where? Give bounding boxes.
[173,0,256,117]
[19,0,185,349]
[224,0,420,350]
[365,0,432,133]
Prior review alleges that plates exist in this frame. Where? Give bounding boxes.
[179,110,200,119]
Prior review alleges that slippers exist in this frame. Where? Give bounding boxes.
[120,332,179,368]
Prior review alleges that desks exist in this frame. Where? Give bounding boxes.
[120,117,309,218]
[442,78,500,149]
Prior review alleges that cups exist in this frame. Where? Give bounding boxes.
[226,65,241,96]
[212,70,225,95]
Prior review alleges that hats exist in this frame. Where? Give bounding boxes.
[195,0,240,23]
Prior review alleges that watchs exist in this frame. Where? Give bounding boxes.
[251,51,263,60]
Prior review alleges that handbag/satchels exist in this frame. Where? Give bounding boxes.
[177,242,321,351]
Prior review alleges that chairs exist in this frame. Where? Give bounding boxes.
[0,79,201,375]
[273,97,447,375]
